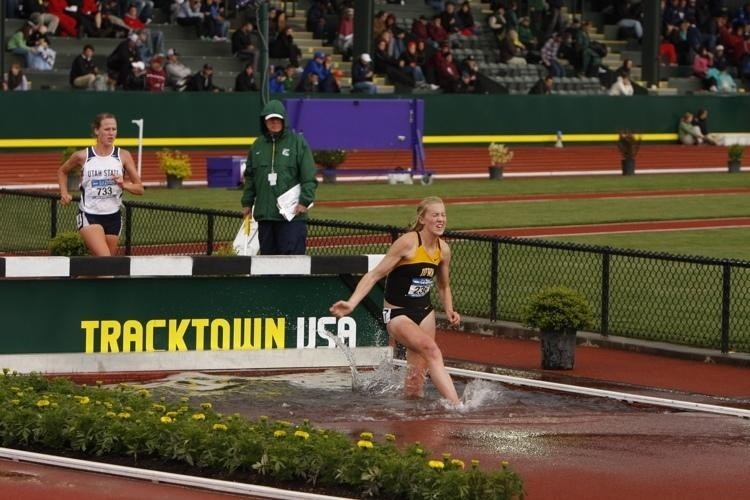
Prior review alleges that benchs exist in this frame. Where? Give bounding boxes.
[0,7,354,96]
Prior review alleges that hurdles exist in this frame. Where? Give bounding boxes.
[0,254,394,377]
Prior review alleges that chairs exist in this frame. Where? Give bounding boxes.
[394,15,606,96]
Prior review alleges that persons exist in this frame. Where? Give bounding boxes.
[241,99,319,255]
[329,197,461,406]
[679,108,723,146]
[372,0,645,96]
[659,1,749,93]
[57,112,145,257]
[2,0,257,91]
[267,1,379,94]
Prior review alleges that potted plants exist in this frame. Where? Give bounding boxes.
[313,149,348,183]
[486,142,515,180]
[615,127,642,175]
[522,282,603,369]
[59,146,83,191]
[727,143,744,173]
[156,147,193,189]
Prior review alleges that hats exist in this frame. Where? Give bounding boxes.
[361,53,371,62]
[129,33,139,43]
[264,113,283,121]
[314,51,326,59]
